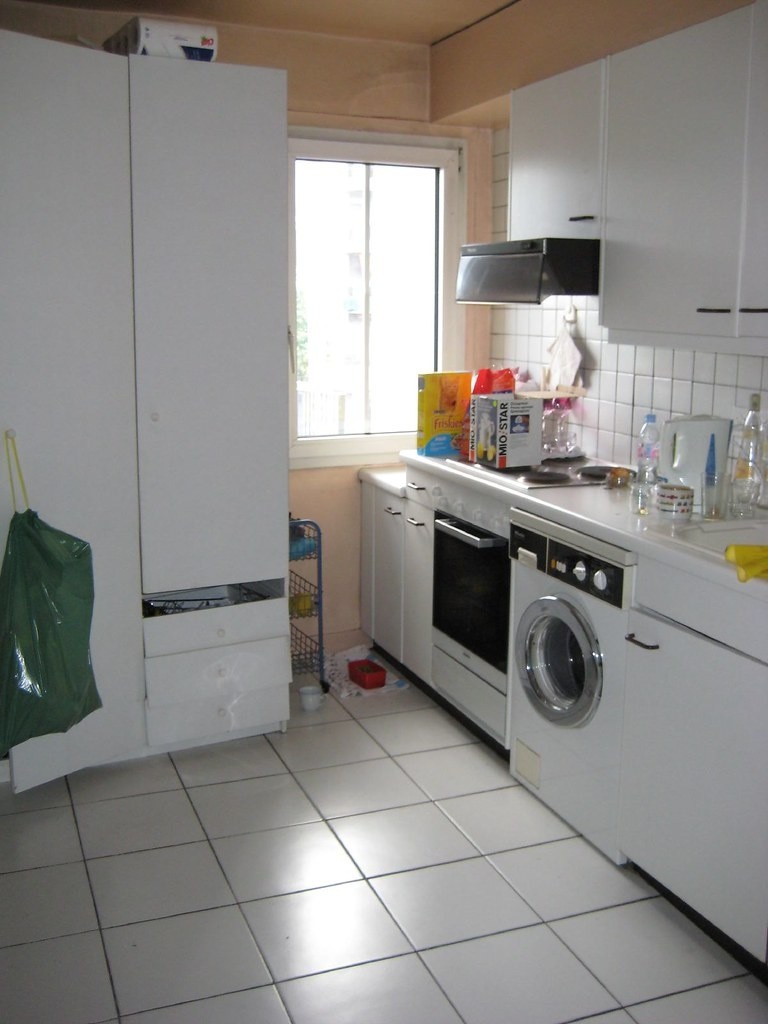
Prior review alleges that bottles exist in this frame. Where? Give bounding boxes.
[734,393,768,509]
[635,414,658,504]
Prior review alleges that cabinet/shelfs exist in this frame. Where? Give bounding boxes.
[0,28,146,795]
[619,552,768,965]
[505,55,607,242]
[599,0,768,361]
[359,488,435,685]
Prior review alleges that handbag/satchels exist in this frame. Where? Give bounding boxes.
[0,432,103,761]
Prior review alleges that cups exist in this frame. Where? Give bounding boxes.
[543,432,577,454]
[732,481,761,517]
[299,686,328,711]
[701,472,731,522]
[658,485,693,521]
[611,468,636,489]
[631,485,652,515]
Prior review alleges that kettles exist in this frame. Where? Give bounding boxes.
[659,414,732,514]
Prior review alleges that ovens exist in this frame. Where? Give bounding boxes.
[431,509,510,737]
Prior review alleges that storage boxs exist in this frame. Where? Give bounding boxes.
[468,383,589,469]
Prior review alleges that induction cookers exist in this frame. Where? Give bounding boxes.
[446,456,636,489]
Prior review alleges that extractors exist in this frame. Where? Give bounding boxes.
[456,238,600,306]
